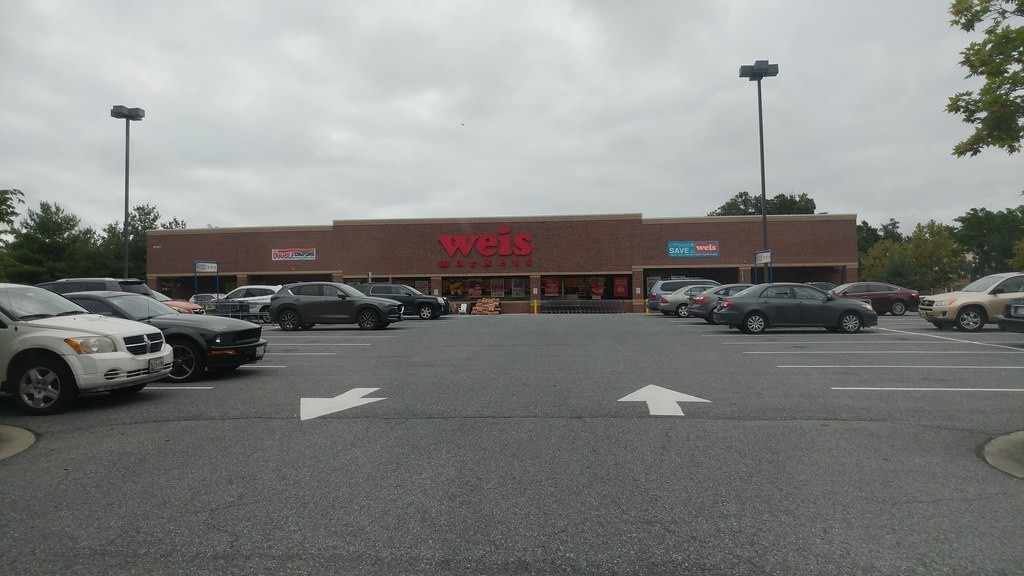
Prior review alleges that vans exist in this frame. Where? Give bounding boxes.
[647,280,721,315]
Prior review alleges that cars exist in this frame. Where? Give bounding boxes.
[998,300,1024,332]
[61,291,268,383]
[189,294,217,310]
[805,282,838,292]
[0,282,174,415]
[151,290,206,315]
[826,282,921,316]
[658,285,716,318]
[713,283,878,333]
[686,284,756,325]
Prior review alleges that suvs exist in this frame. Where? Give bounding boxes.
[362,284,451,320]
[917,272,1024,332]
[34,278,155,299]
[207,285,283,323]
[269,281,404,331]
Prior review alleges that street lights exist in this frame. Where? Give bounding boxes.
[739,61,778,282]
[111,105,145,277]
